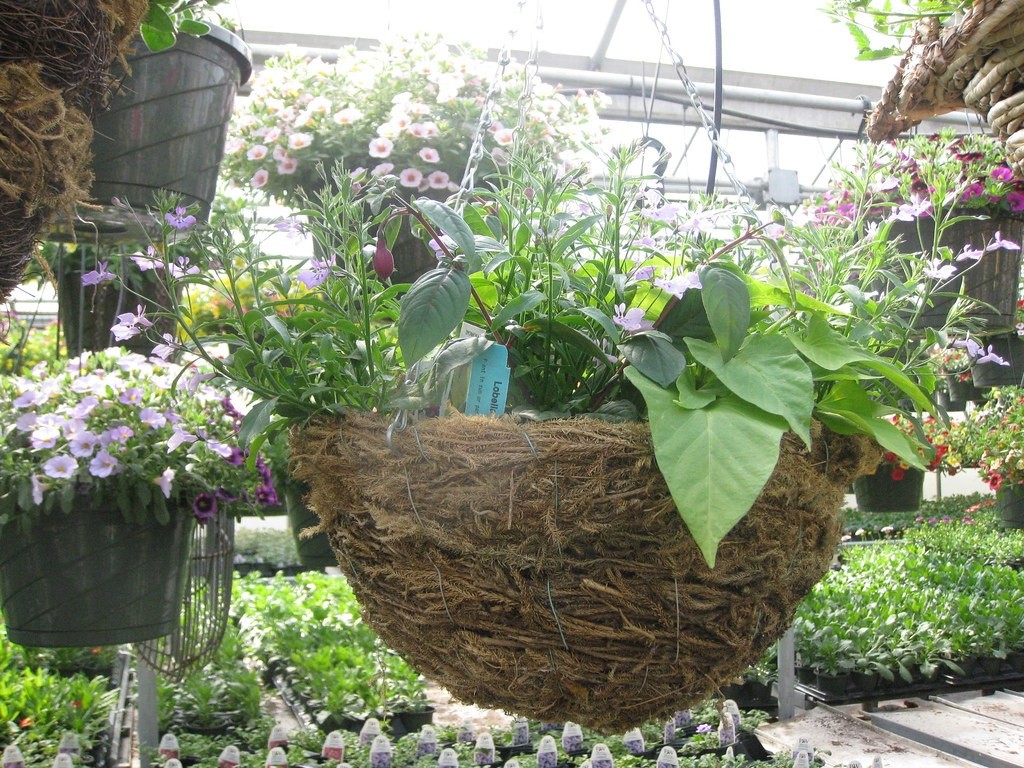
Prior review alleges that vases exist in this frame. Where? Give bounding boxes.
[59,249,181,362]
[853,460,923,512]
[284,405,875,740]
[0,503,196,647]
[867,0,1023,187]
[46,21,253,242]
[996,485,1024,529]
[0,1,155,308]
[302,180,505,300]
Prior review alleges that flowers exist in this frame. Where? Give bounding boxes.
[217,28,612,194]
[0,566,812,768]
[841,493,995,546]
[80,67,1024,570]
[880,385,1024,491]
[0,345,282,529]
